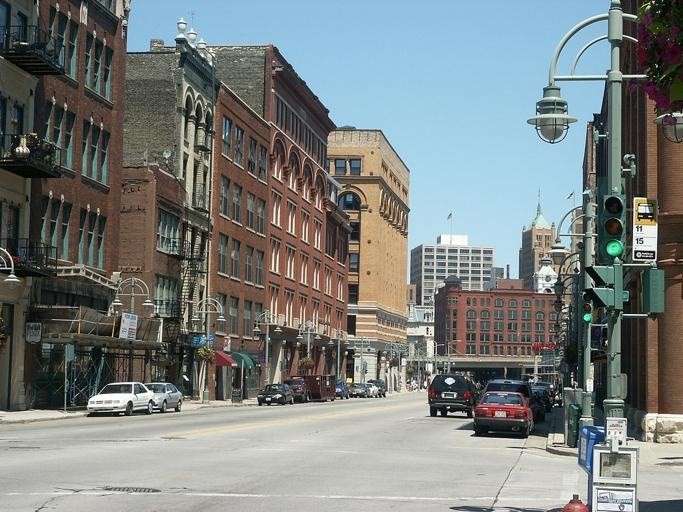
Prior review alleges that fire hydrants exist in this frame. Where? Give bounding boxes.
[562,493,591,512]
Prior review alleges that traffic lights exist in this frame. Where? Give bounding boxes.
[582,260,625,316]
[649,269,665,318]
[578,289,598,326]
[599,186,628,259]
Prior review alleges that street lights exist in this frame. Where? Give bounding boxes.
[551,179,607,427]
[252,305,283,388]
[192,295,229,405]
[297,316,456,393]
[1,248,23,290]
[115,274,157,384]
[544,243,584,409]
[521,0,683,446]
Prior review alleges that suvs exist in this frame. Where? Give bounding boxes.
[426,371,476,419]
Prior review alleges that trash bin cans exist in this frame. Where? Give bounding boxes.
[567,403,595,448]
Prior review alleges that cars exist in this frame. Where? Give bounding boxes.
[142,382,186,416]
[87,377,160,418]
[467,376,557,440]
[257,369,389,408]
